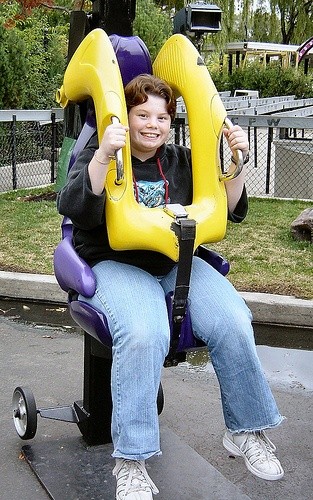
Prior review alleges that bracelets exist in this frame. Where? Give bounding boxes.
[93,148,113,165]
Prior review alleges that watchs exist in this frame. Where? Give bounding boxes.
[231,151,250,165]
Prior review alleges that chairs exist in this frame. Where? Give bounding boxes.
[55,26,245,368]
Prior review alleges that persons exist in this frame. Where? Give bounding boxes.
[55,73,286,500]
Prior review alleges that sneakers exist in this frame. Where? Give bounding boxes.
[113,456,159,499]
[221,430,284,480]
